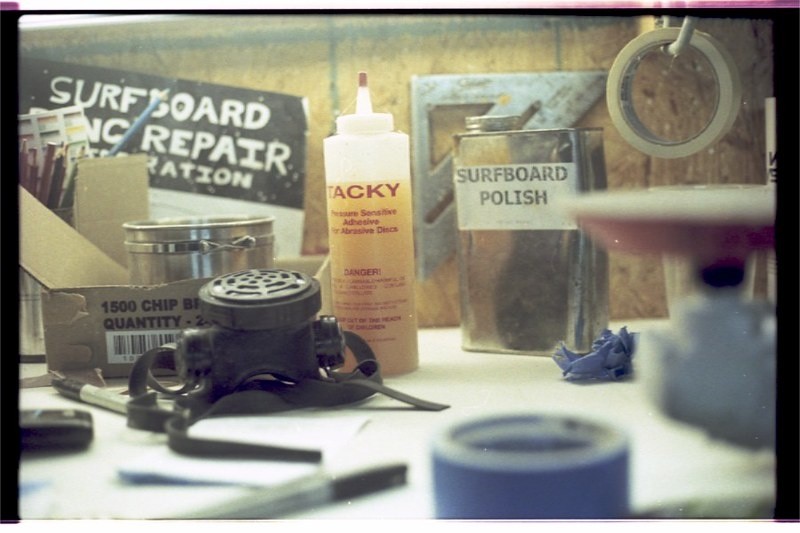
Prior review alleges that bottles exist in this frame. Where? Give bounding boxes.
[318,72,421,379]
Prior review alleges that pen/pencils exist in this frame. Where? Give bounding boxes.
[178,462,410,519]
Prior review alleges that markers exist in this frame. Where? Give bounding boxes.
[52,377,129,415]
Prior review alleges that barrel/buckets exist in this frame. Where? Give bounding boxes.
[125,210,273,292]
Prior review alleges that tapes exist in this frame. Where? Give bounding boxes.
[604,26,743,159]
[431,413,632,519]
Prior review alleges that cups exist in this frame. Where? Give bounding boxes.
[434,415,628,518]
